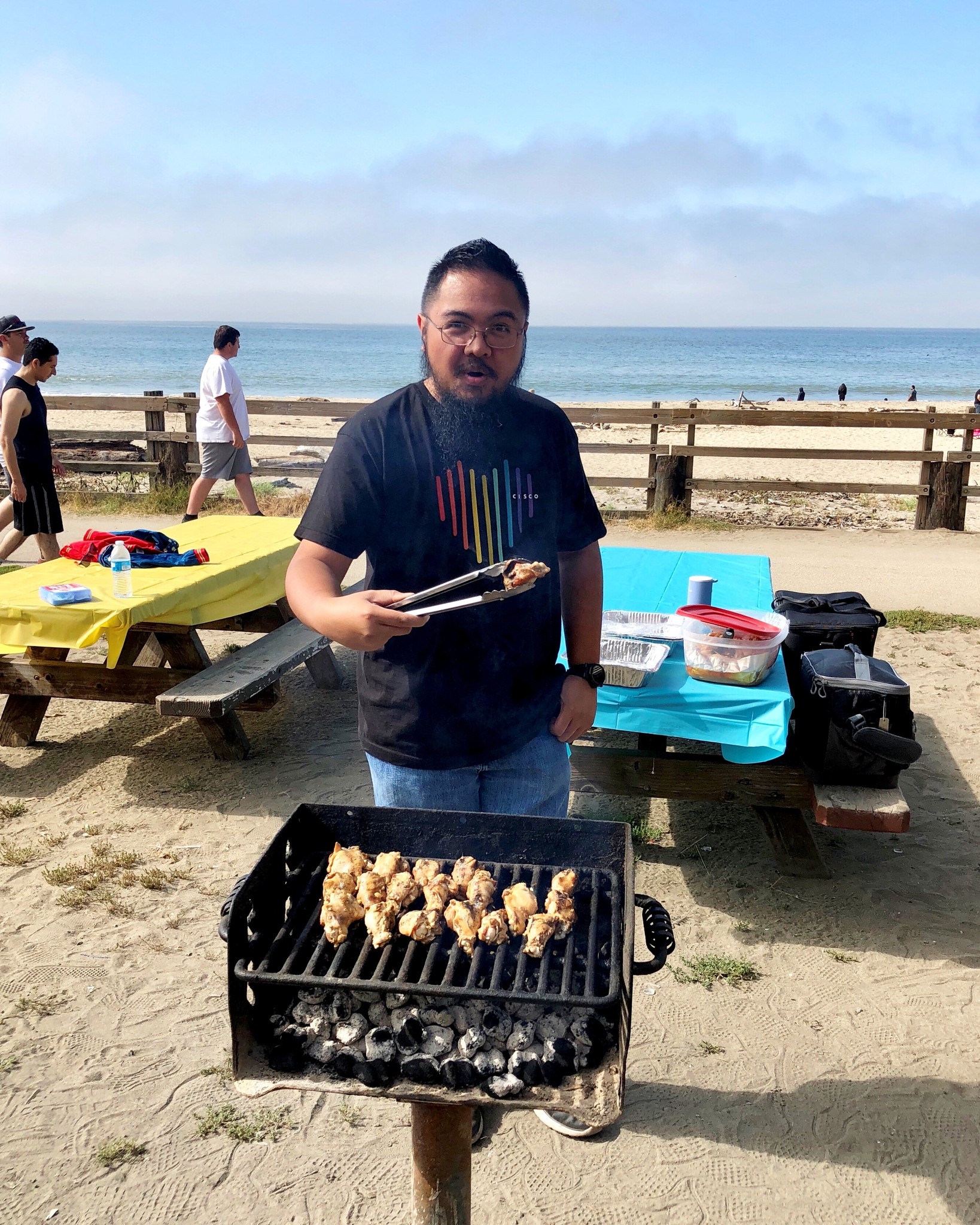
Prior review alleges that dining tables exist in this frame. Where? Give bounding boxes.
[555,546,833,880]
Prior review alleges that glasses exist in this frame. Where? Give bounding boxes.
[1,321,26,334]
[422,312,526,348]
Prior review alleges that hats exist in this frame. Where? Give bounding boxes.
[0,314,35,335]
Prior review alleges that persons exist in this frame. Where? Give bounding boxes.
[884,398,888,401]
[283,239,607,1153]
[798,387,805,400]
[907,385,917,401]
[838,383,847,401]
[0,315,35,534]
[180,325,267,523]
[974,389,980,413]
[0,338,64,562]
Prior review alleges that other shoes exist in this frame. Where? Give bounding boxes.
[470,1107,482,1143]
[536,1110,603,1138]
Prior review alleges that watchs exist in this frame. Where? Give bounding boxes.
[567,662,606,689]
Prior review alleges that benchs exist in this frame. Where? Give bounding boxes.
[794,769,910,833]
[156,578,365,717]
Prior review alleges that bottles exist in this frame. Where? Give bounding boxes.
[111,540,132,599]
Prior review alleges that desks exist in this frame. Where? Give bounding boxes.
[0,515,347,758]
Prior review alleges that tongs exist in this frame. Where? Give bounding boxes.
[385,559,535,618]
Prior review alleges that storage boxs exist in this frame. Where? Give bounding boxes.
[676,604,790,687]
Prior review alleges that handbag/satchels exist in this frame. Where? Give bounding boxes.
[795,645,911,788]
[773,590,888,674]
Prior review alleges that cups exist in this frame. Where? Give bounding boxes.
[687,576,719,606]
